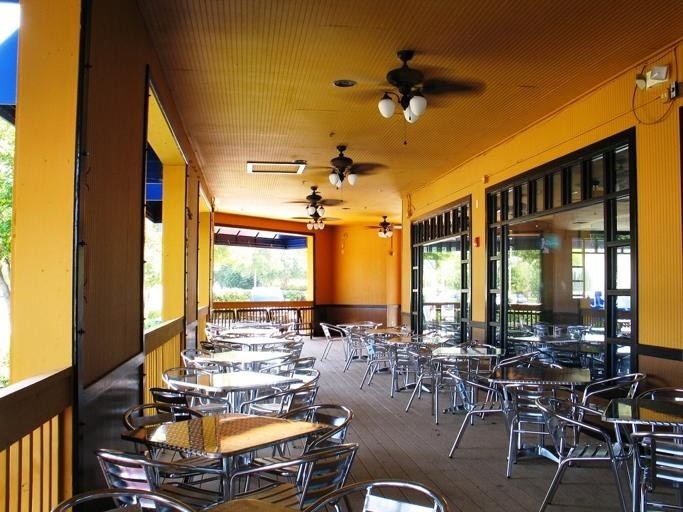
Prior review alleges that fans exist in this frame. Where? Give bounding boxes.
[330,49,490,108]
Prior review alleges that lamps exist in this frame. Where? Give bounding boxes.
[375,51,429,124]
[281,142,402,241]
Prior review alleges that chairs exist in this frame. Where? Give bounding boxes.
[54,317,453,512]
[318,317,681,511]
